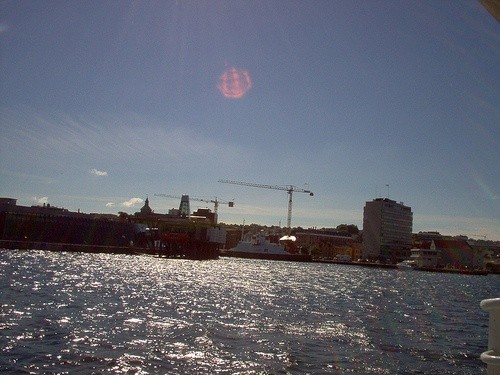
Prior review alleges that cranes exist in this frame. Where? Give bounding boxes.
[154,193,234,226]
[218,179,313,229]
